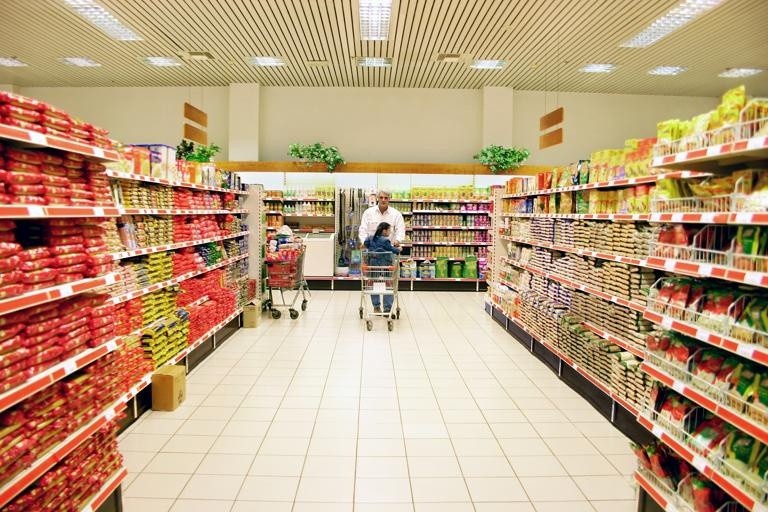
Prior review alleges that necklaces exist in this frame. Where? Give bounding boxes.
[379,207,387,214]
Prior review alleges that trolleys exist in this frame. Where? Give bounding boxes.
[357,244,402,333]
[265,237,311,319]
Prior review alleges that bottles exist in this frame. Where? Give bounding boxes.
[282,184,334,200]
[413,202,490,259]
[264,202,333,216]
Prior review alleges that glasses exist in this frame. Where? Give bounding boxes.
[379,196,389,200]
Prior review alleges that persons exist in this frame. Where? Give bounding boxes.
[364,222,403,286]
[358,189,406,317]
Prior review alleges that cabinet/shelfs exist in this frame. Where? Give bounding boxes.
[254,182,495,295]
[622,113,768,512]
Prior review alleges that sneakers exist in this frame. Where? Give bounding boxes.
[383,311,390,317]
[374,307,382,316]
